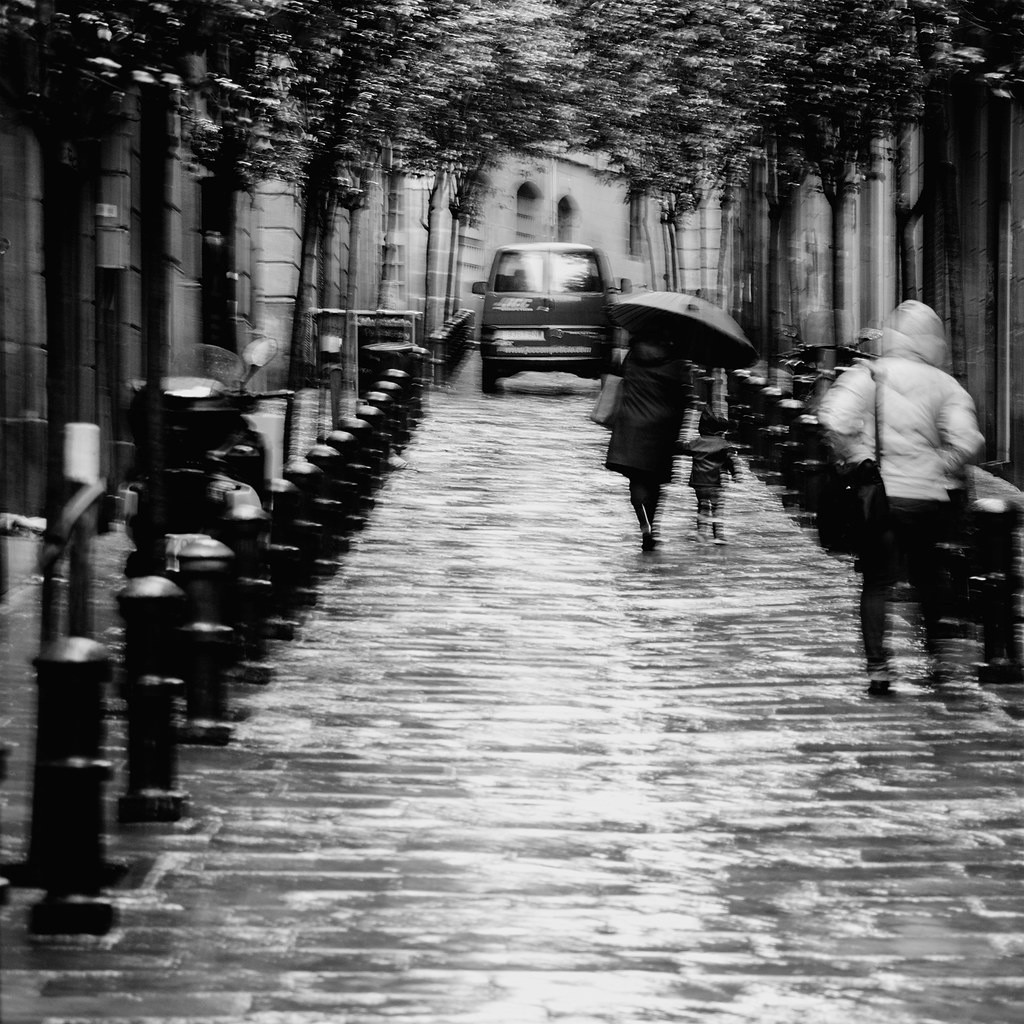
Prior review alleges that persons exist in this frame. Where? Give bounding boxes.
[679,407,742,543]
[601,314,692,550]
[820,298,986,695]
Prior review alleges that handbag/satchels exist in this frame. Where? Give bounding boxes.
[815,459,891,556]
[590,347,632,426]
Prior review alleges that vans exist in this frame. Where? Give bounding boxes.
[472,240,617,393]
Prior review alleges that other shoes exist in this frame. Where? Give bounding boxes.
[866,672,940,695]
[698,537,728,546]
[641,531,668,552]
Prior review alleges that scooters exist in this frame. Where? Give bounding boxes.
[779,316,881,412]
[118,332,280,584]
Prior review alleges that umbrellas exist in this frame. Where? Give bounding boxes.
[601,287,762,369]
